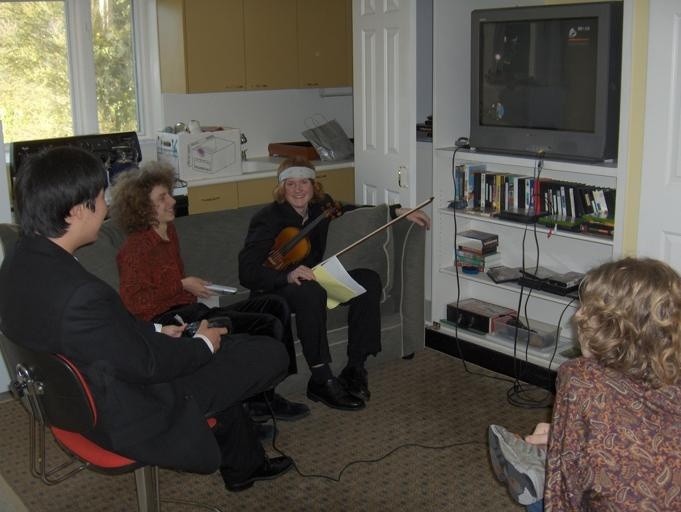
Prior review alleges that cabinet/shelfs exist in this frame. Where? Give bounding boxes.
[297,0,347,89]
[154,0,298,94]
[315,168,355,204]
[433,147,617,375]
[188,181,238,214]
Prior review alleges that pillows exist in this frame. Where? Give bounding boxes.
[327,203,396,305]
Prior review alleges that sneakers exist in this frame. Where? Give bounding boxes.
[488,424,548,506]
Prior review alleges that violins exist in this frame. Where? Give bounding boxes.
[260,199,344,273]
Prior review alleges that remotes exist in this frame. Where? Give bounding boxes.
[204,283,237,295]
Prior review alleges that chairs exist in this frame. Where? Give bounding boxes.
[1,331,217,511]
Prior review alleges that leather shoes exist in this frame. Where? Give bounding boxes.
[253,394,310,422]
[221,456,293,491]
[251,423,277,443]
[339,366,370,401]
[307,374,365,411]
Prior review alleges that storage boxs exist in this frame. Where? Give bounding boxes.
[155,124,242,181]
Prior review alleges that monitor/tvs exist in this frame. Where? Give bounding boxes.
[466,2,617,164]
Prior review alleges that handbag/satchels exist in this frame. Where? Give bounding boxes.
[302,114,354,161]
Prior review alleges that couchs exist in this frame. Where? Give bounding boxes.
[79,202,425,379]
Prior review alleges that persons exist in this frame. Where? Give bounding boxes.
[109,159,309,423]
[488,255,681,512]
[237,154,431,412]
[0,146,295,493]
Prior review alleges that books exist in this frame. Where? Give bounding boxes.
[455,229,502,272]
[454,163,616,240]
